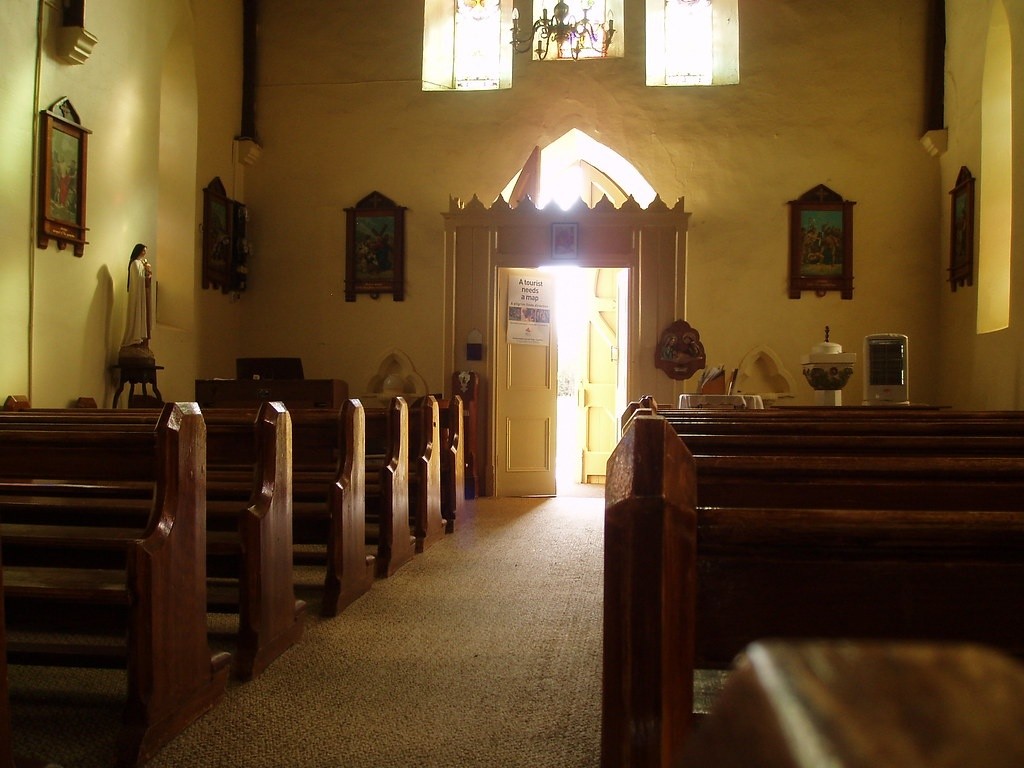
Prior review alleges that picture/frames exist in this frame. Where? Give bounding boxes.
[36,95,93,258]
[342,190,409,302]
[551,221,579,260]
[786,183,857,300]
[200,176,233,295]
[945,165,977,293]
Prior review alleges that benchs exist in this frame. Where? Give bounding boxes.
[598,401,1024,768]
[0,394,469,768]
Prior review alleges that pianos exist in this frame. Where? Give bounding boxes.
[196,357,349,411]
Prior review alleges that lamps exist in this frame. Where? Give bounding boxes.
[510,0,617,62]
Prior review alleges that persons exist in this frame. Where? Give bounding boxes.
[119,242,153,350]
